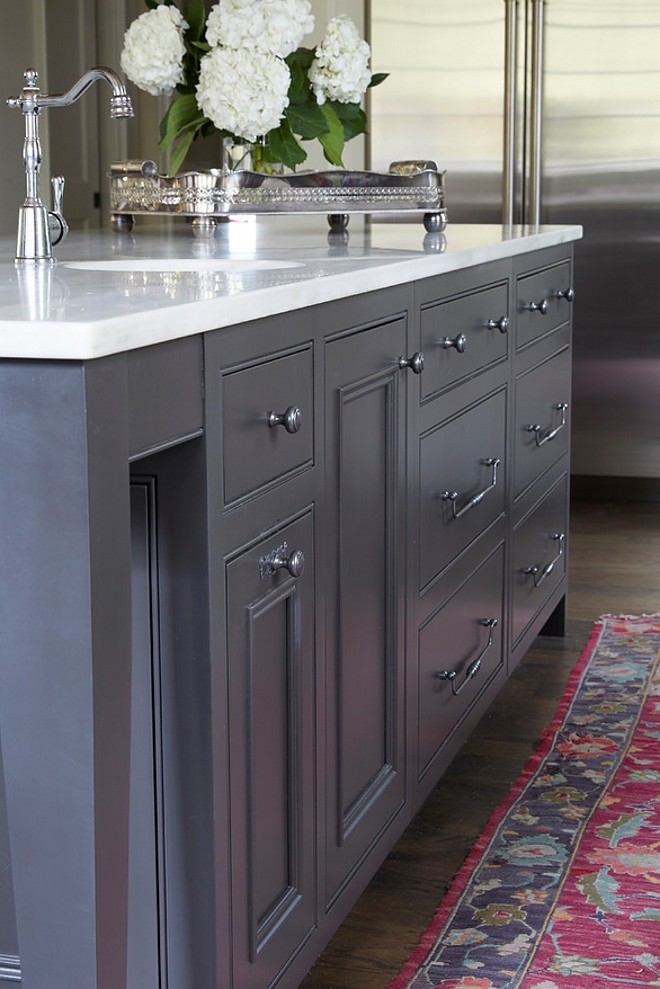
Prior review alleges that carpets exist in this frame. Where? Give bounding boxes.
[387,609,660,989]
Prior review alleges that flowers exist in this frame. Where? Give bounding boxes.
[122,0,389,174]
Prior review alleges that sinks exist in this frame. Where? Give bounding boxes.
[63,257,307,276]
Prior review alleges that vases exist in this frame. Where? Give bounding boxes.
[223,135,284,174]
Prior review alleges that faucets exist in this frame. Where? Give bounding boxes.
[6,65,138,261]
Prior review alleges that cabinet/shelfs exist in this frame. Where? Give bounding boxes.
[1,246,573,989]
